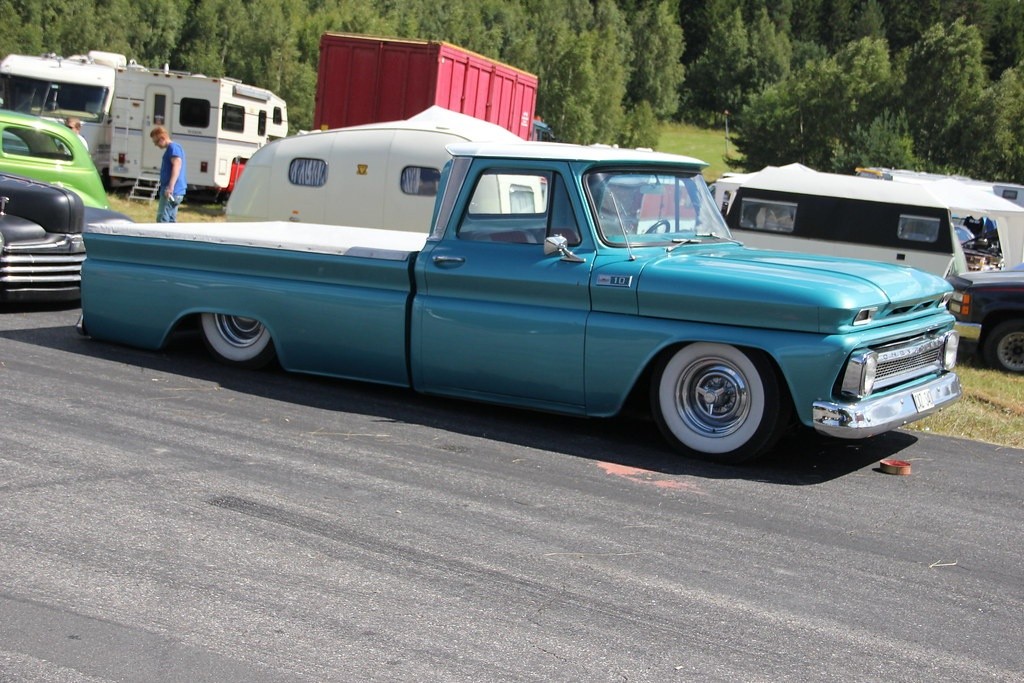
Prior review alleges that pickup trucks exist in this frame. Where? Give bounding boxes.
[77,143,963,463]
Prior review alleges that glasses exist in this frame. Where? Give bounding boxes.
[154,138,161,144]
[65,126,73,129]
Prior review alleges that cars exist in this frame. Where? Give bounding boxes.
[0,108,109,210]
[947,264,1024,373]
[0,170,135,302]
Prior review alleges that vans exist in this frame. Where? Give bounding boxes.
[0,50,127,187]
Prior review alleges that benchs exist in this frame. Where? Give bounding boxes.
[473,228,580,246]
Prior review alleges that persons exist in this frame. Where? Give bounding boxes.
[59,115,91,159]
[149,127,187,223]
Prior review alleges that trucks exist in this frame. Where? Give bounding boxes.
[109,62,288,204]
[313,32,556,142]
